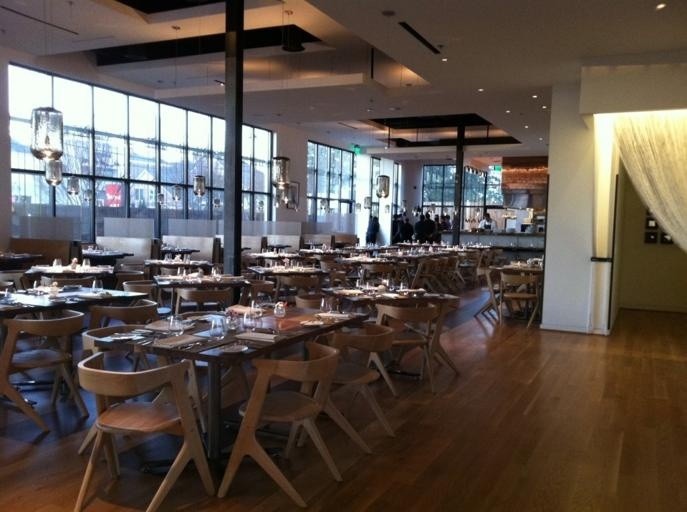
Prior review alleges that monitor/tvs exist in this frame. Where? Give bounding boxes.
[505,217,517,230]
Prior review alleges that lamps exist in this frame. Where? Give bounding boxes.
[30,0,94,202]
[157,25,220,208]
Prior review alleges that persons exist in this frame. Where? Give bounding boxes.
[365,213,452,244]
[476,212,499,234]
